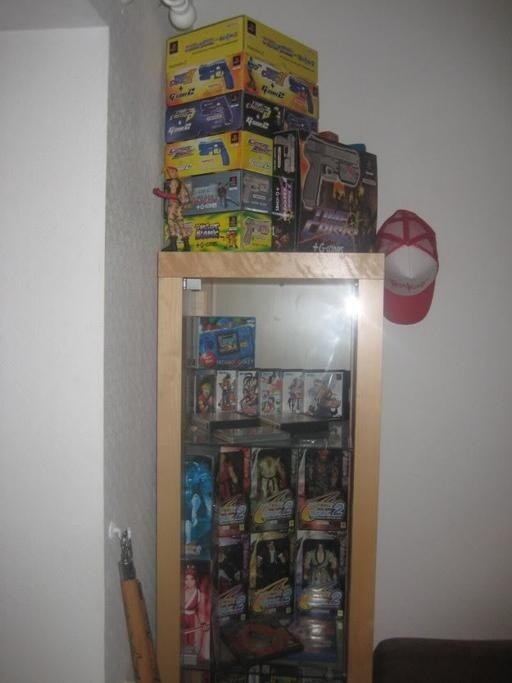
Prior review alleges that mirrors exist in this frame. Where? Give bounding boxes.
[154,249,386,681]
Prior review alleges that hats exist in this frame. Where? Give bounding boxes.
[375,210,437,325]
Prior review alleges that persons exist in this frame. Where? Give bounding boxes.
[302,542,338,588]
[305,449,342,502]
[217,548,241,595]
[256,541,288,587]
[257,453,287,503]
[218,453,238,500]
[183,565,210,648]
